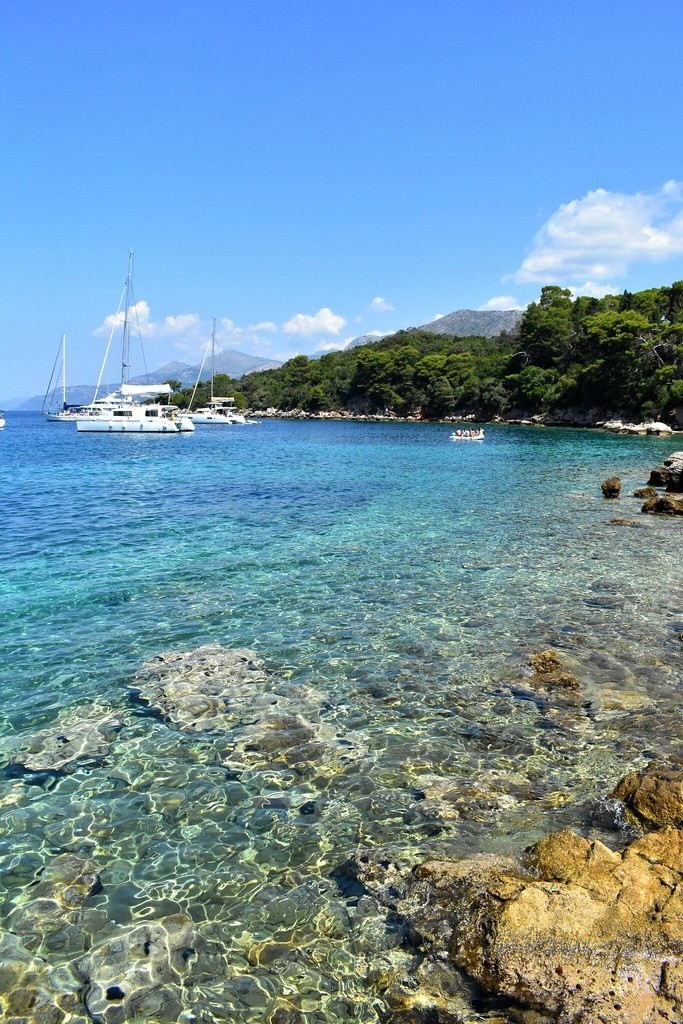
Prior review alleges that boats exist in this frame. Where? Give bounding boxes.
[450,433,486,441]
[247,420,263,424]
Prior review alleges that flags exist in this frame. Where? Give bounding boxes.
[124,364,131,367]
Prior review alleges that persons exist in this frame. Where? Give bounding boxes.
[131,401,160,406]
[456,427,481,437]
[170,414,175,420]
[223,410,227,417]
[89,411,98,416]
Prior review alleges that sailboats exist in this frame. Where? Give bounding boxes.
[39,249,246,434]
